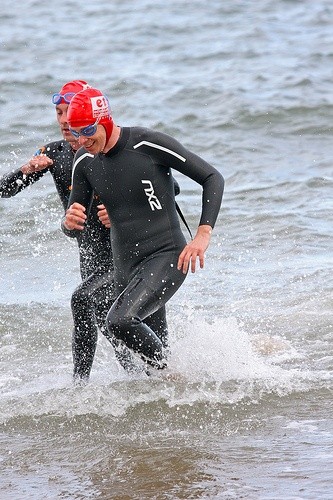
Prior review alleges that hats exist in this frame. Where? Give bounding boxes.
[58,80,90,104]
[66,87,113,152]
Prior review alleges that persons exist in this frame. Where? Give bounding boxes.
[0,79,147,388]
[60,88,225,375]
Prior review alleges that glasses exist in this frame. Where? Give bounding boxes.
[51,93,77,104]
[68,114,102,138]
